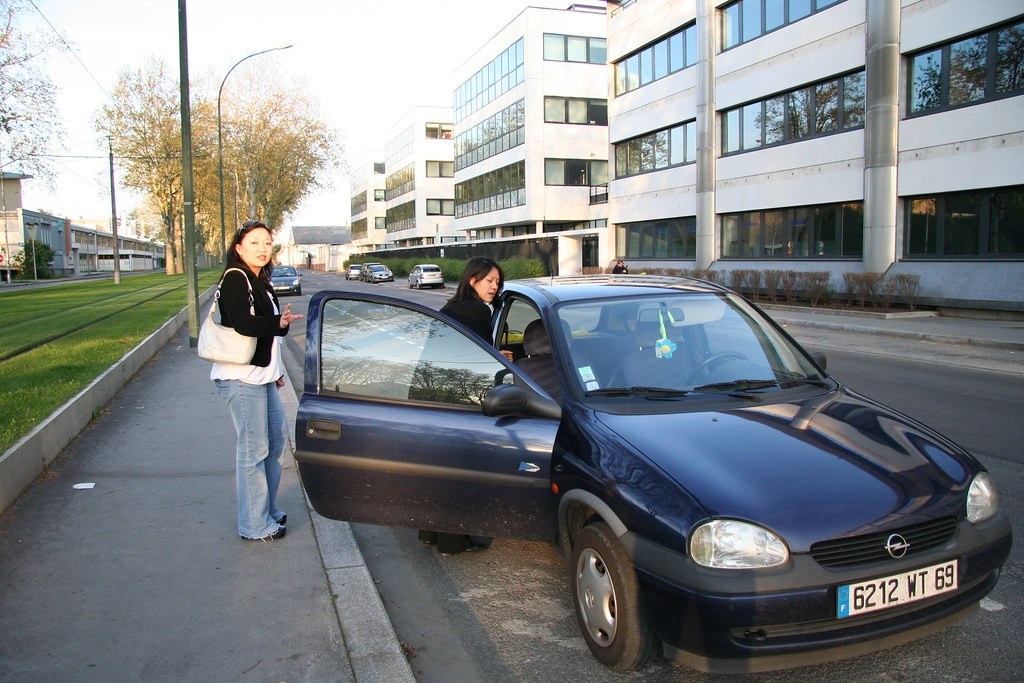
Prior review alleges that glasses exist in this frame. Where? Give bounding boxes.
[238,220,269,235]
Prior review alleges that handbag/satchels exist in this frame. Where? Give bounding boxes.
[197,268,257,365]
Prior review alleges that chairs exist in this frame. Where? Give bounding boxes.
[623,320,692,389]
[513,318,588,407]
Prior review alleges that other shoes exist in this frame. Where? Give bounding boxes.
[441,542,486,556]
[423,537,438,544]
[276,515,287,525]
[242,526,286,540]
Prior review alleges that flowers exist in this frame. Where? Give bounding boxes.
[655,340,677,360]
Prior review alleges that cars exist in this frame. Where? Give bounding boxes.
[345,264,363,280]
[366,265,394,283]
[408,263,445,290]
[269,265,303,296]
[293,273,1014,674]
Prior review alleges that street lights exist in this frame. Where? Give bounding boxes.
[218,43,295,274]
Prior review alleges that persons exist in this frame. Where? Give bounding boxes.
[612,259,628,274]
[407,256,505,557]
[211,222,306,542]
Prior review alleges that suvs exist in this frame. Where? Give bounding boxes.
[358,262,384,282]
